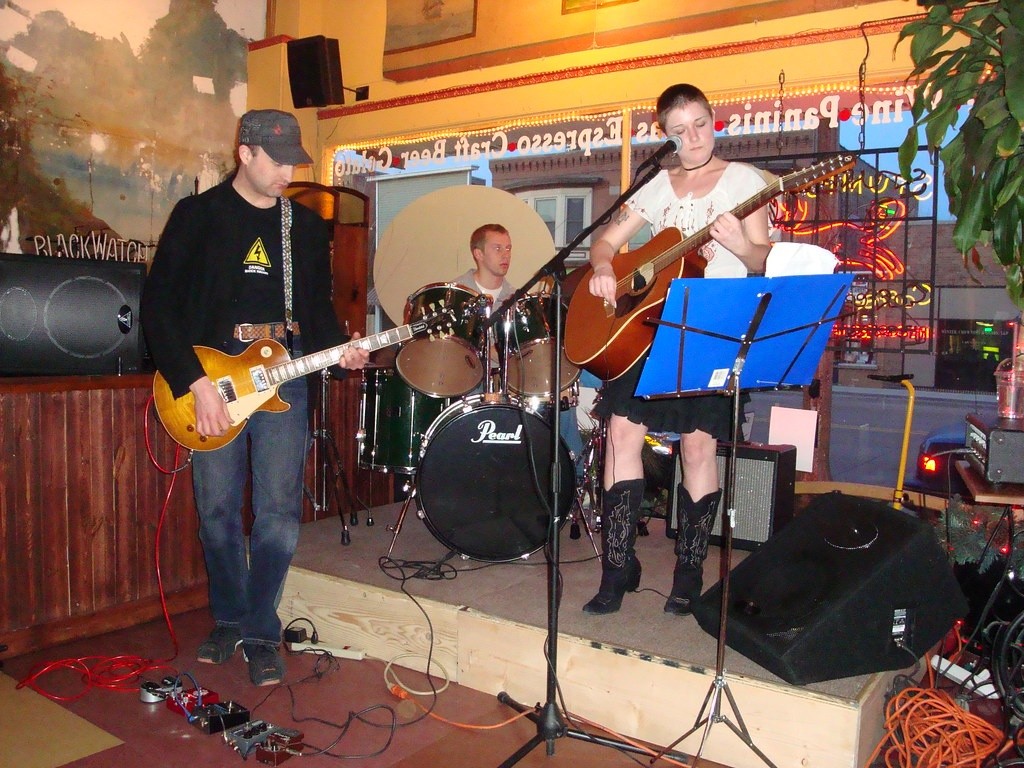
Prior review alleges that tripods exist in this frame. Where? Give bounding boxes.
[302,366,361,547]
[494,171,858,768]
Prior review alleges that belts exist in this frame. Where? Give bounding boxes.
[233,322,299,343]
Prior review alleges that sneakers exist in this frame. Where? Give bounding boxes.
[243,641,285,687]
[197,626,243,664]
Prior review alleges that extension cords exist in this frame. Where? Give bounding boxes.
[292,639,367,661]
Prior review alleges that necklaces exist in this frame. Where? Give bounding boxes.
[682,154,713,171]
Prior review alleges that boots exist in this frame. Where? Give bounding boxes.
[583,479,645,616]
[663,483,723,616]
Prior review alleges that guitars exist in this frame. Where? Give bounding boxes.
[153,299,459,451]
[564,153,861,383]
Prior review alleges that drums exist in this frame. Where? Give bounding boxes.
[412,393,577,564]
[356,364,445,475]
[493,291,583,397]
[395,281,484,400]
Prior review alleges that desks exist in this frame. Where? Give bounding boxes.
[934,461,1024,768]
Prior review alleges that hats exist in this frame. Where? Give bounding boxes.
[238,110,313,165]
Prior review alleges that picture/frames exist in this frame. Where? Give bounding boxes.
[383,0,478,56]
[561,0,640,15]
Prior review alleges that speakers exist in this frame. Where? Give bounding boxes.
[287,35,346,109]
[666,439,798,548]
[0,252,147,377]
[693,492,969,687]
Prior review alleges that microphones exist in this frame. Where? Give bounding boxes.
[637,135,683,173]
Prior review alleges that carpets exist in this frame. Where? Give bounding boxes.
[0,670,125,768]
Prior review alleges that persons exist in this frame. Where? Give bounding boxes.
[447,224,607,515]
[582,84,772,616]
[140,109,370,686]
